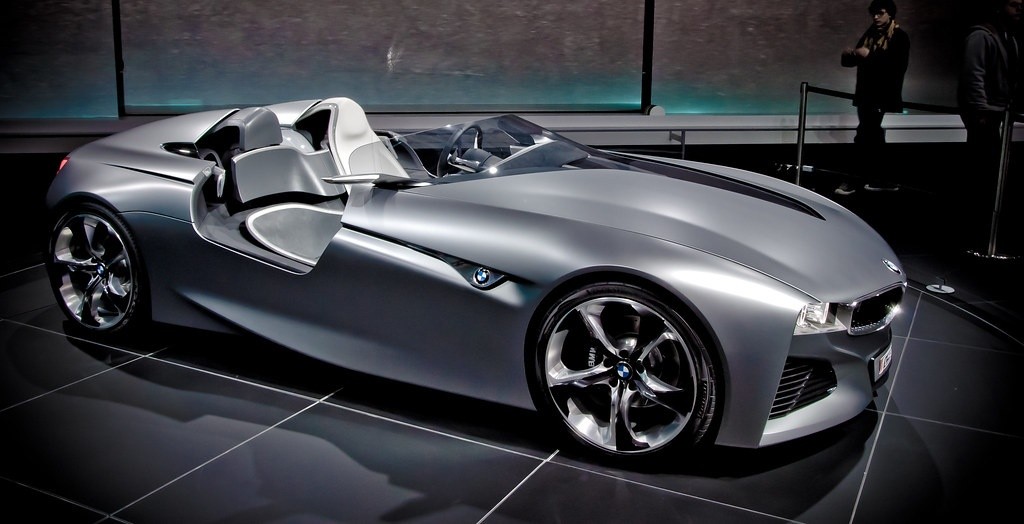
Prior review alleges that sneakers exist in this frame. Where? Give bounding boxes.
[863,181,898,191]
[835,182,855,195]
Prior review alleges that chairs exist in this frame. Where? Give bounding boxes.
[224,97,409,267]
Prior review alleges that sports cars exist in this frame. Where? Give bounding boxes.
[43,98,908,465]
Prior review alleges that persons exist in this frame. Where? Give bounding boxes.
[963,0,1024,224]
[834,0,910,195]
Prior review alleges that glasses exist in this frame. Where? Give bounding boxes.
[871,11,888,17]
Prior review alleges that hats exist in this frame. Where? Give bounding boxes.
[868,0,896,17]
